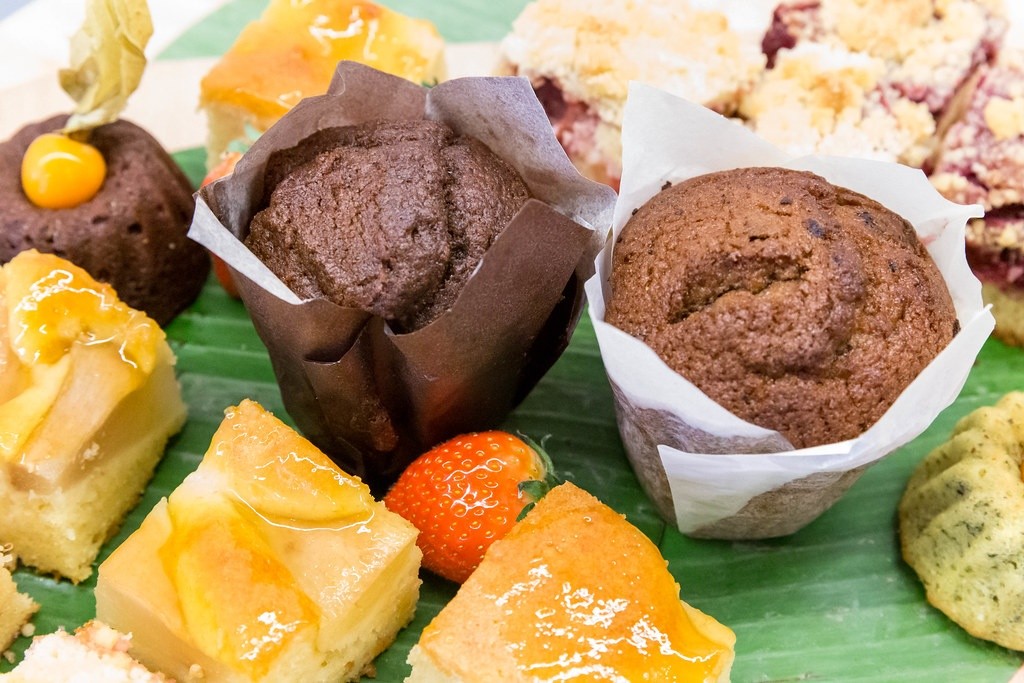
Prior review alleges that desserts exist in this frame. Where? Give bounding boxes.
[1,1,1024,683]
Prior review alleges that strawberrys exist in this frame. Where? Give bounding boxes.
[381,426,572,585]
